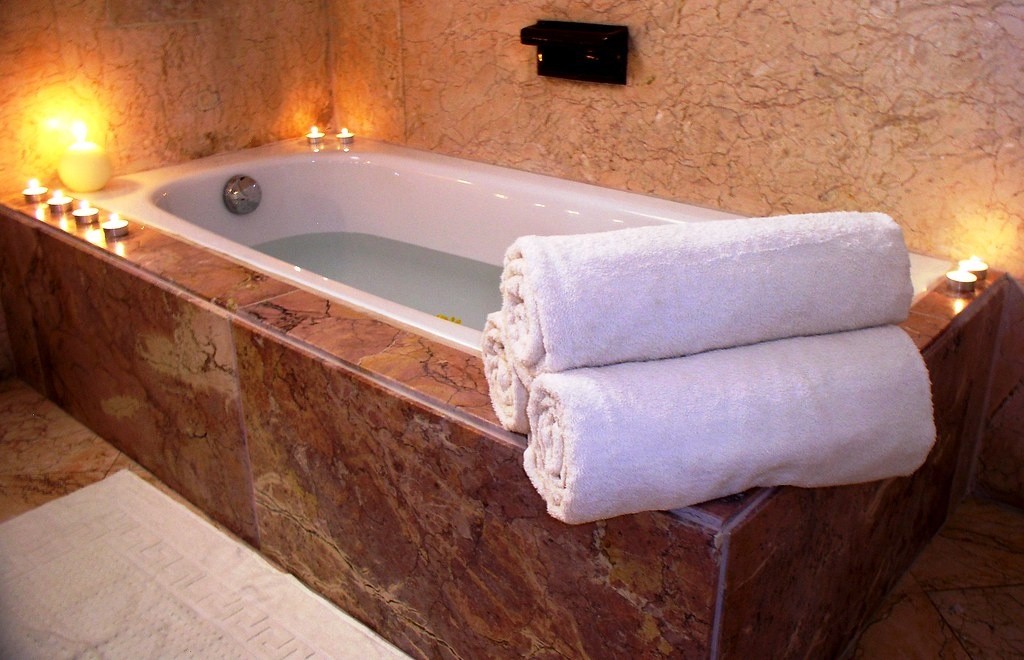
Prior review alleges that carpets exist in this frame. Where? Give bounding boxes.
[0,470,418,660]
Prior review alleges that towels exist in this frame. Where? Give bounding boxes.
[476,205,939,528]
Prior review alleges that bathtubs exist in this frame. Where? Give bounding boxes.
[51,127,1011,659]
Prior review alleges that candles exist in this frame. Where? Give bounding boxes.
[306,126,325,144]
[46,190,72,213]
[23,177,45,204]
[102,213,130,241]
[945,267,979,291]
[335,127,354,146]
[55,120,115,194]
[71,200,97,225]
[958,256,989,279]
